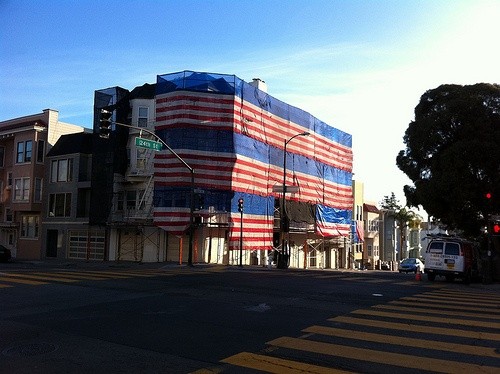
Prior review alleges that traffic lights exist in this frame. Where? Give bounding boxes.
[98,109,112,139]
[238,199,243,212]
[489,220,500,237]
[196,216,204,223]
[282,217,291,232]
[484,184,493,208]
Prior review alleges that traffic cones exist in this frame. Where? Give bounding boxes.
[415,265,422,282]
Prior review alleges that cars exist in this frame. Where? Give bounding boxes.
[398,258,425,274]
[0,244,11,262]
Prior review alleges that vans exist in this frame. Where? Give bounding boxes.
[423,239,482,284]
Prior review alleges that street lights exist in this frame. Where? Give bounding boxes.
[282,131,311,254]
